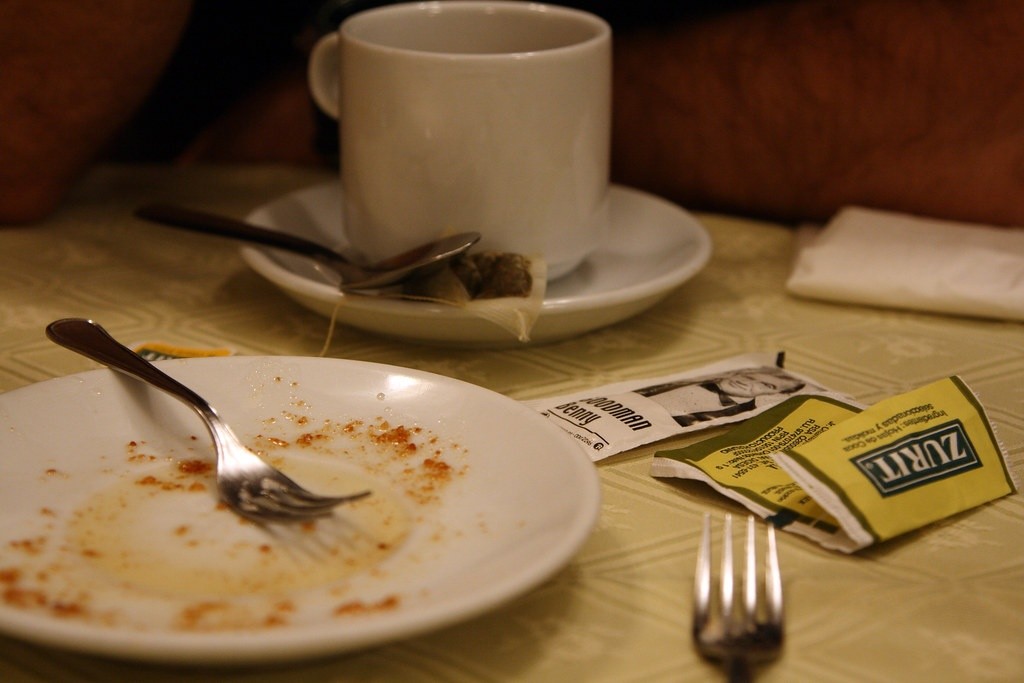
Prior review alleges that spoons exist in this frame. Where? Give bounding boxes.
[132,198,484,291]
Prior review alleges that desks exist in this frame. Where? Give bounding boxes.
[0,165,1024,681]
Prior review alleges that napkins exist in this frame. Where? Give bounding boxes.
[785,206,1023,321]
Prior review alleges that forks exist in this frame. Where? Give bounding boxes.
[45,317,372,517]
[692,512,784,683]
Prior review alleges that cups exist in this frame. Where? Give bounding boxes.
[307,0,610,280]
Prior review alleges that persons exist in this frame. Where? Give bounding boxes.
[0,1,1024,228]
[632,367,806,427]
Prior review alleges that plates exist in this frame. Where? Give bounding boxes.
[240,182,713,349]
[0,355,599,669]
